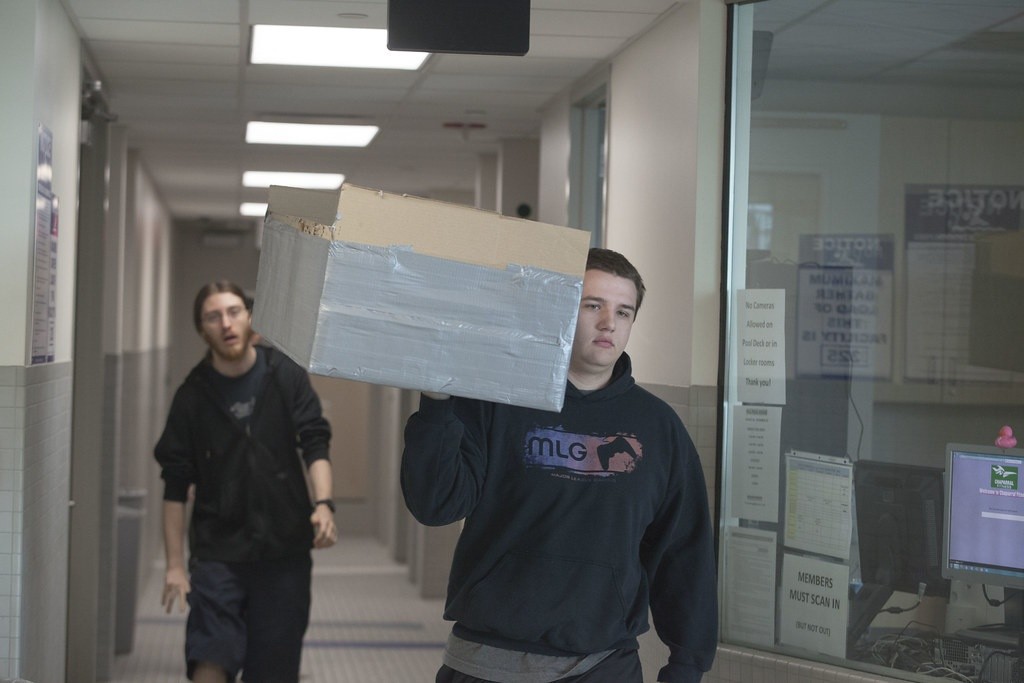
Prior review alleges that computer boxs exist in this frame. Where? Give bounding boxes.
[925,633,1024,683]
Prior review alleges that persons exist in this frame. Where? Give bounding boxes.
[399,246,720,683]
[152,279,338,683]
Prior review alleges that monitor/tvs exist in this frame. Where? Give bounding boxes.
[845,460,951,655]
[942,443,1024,589]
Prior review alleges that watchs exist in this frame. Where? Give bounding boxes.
[314,497,337,514]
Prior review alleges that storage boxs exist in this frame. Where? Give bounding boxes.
[249,181,591,413]
